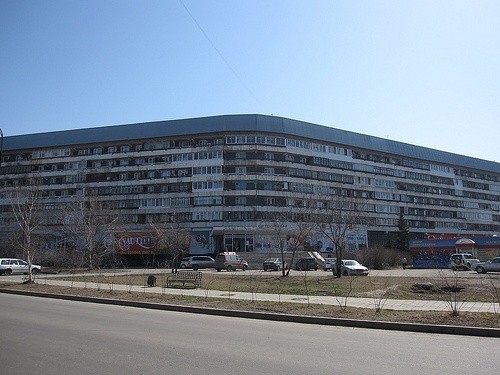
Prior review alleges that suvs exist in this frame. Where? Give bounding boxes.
[450,253,479,271]
[296,257,318,271]
[323,258,337,270]
[188,256,215,270]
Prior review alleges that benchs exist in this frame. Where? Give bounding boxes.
[167,269,204,288]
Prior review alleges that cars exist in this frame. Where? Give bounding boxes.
[263,258,289,271]
[331,260,369,276]
[472,257,500,273]
[180,257,193,268]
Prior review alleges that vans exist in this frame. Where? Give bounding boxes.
[215,252,248,272]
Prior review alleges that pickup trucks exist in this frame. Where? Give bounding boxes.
[0,258,41,275]
